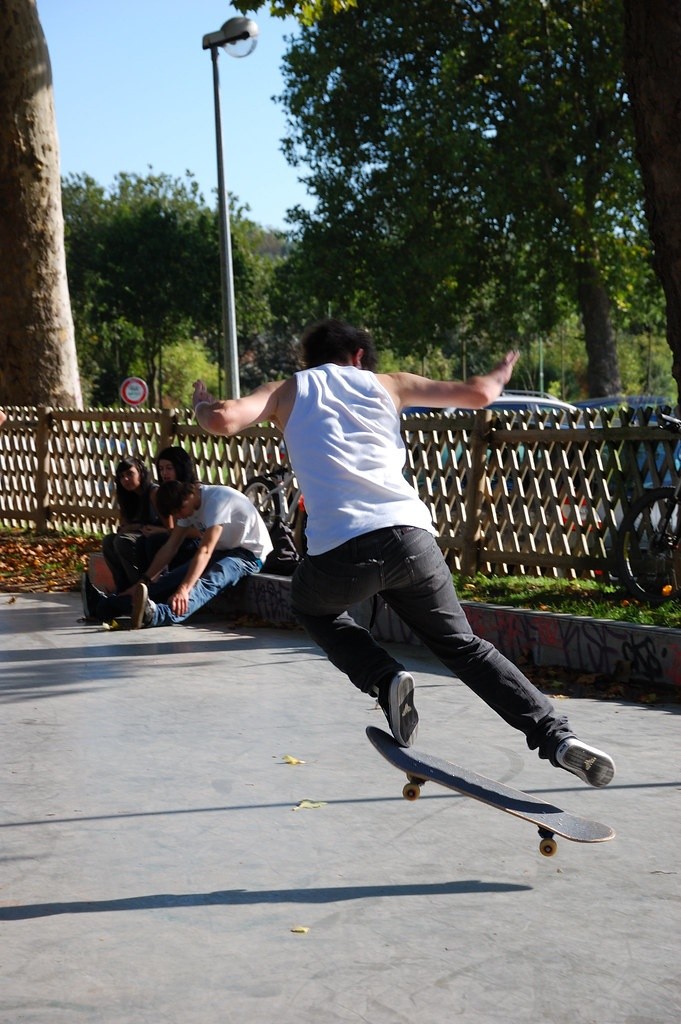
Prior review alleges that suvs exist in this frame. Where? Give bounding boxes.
[396,388,672,490]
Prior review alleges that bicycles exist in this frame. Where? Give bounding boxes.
[238,447,308,558]
[615,413,679,608]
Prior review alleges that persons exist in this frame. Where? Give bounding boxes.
[80,445,206,625]
[101,456,161,595]
[191,318,618,789]
[118,479,278,630]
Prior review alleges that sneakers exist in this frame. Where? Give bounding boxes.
[375,670,419,748]
[80,572,105,621]
[554,737,616,788]
[130,582,155,630]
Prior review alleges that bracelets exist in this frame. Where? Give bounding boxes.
[141,573,156,587]
[195,400,211,413]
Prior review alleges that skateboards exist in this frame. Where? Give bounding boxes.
[366,726,615,855]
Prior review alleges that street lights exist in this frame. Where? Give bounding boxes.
[202,18,257,406]
